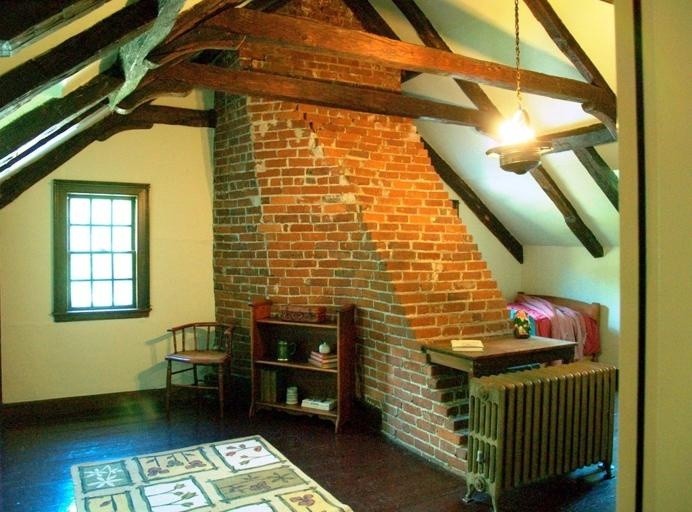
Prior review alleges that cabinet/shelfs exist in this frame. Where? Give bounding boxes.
[247,299,356,433]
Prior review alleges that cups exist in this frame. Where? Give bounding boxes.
[276,338,296,361]
[286,386,299,404]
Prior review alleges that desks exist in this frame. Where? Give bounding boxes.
[423,334,579,382]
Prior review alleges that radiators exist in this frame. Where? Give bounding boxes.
[463,361,617,512]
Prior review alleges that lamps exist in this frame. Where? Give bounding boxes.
[486,0,556,175]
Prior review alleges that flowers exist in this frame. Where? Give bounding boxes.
[514,311,531,333]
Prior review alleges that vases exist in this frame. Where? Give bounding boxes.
[516,325,529,338]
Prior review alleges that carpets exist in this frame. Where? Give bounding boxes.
[71,434,353,512]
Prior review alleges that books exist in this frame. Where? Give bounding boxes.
[260,367,287,403]
[311,350,337,360]
[451,339,485,352]
[307,357,337,368]
[309,354,337,364]
[301,396,336,411]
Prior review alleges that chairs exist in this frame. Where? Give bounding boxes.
[165,321,234,419]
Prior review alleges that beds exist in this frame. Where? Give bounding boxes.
[507,291,602,363]
